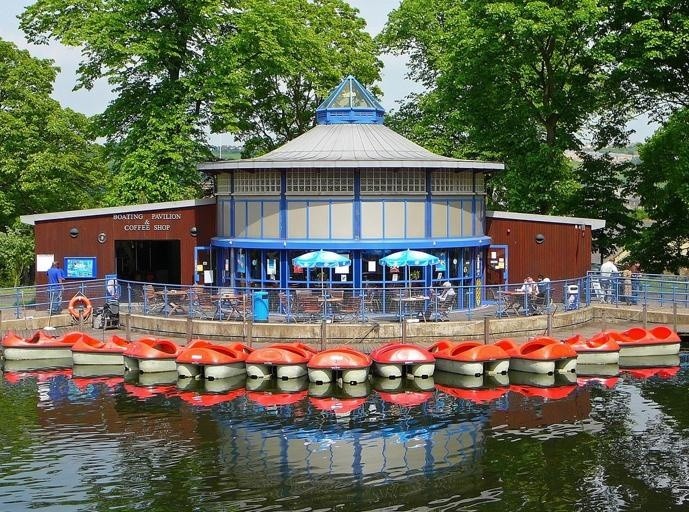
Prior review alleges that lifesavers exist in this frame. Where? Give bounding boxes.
[68,296,91,320]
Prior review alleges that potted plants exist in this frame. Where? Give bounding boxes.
[411,269,421,286]
[313,266,328,281]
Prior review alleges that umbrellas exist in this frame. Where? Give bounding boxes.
[292,250,351,295]
[378,248,440,297]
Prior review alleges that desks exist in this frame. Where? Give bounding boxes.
[390,296,430,322]
[497,290,532,319]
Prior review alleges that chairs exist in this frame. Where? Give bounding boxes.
[431,294,457,321]
[278,290,382,324]
[489,288,511,317]
[532,287,555,316]
[139,284,252,322]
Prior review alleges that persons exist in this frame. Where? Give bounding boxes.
[46,261,66,314]
[600,258,640,306]
[515,275,551,316]
[420,282,455,321]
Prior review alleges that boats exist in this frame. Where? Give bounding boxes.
[432,369,512,404]
[576,363,623,390]
[617,353,682,380]
[0,330,105,360]
[69,333,158,366]
[425,340,510,375]
[174,373,248,408]
[245,343,319,382]
[306,346,374,385]
[307,381,370,424]
[245,374,308,411]
[604,325,682,356]
[0,357,72,385]
[123,369,178,402]
[508,370,579,401]
[369,375,435,415]
[558,333,621,365]
[122,338,184,372]
[370,340,436,378]
[175,340,256,395]
[494,336,578,375]
[71,362,125,395]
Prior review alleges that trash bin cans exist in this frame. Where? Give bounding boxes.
[564,285,579,311]
[253,292,269,323]
